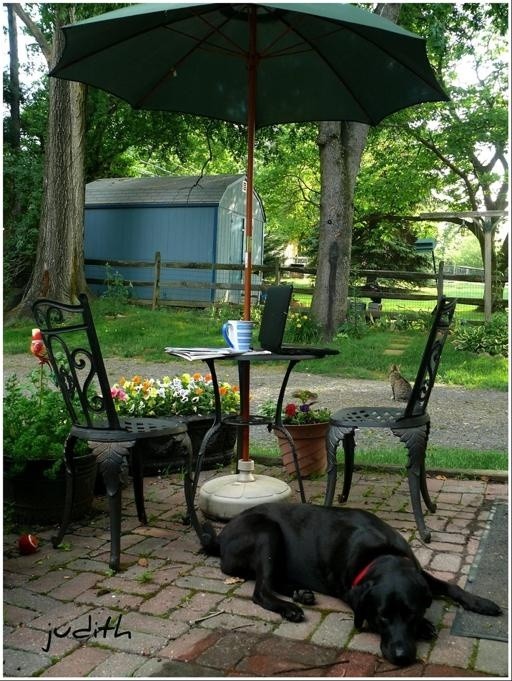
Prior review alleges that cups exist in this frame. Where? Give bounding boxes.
[222,319,255,352]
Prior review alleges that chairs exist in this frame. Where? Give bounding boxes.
[323,295,461,543]
[32,290,194,568]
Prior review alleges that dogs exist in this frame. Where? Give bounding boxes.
[196,498,503,669]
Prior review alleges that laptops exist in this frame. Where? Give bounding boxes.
[259,285,341,357]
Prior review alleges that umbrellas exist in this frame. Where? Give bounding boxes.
[43,4,459,462]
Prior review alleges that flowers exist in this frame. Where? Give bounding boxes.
[94,370,252,417]
[3,326,93,478]
[257,388,337,432]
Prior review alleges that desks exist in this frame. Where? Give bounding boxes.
[165,345,342,557]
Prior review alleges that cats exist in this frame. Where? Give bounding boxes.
[388,362,414,404]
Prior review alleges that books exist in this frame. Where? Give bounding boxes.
[165,344,272,362]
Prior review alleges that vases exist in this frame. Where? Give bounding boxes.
[127,412,239,477]
[273,420,328,480]
[2,447,98,527]
